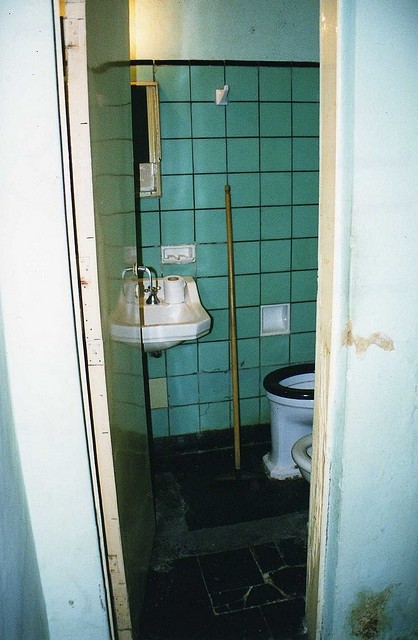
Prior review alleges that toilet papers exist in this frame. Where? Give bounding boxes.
[163,275,186,304]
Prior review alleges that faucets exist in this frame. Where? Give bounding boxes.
[143,286,160,305]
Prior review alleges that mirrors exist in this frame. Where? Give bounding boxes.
[130,82,161,198]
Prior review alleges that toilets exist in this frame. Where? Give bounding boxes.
[290,433,312,483]
[263,363,315,480]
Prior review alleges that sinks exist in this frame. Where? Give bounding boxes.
[143,275,211,353]
[109,275,141,349]
